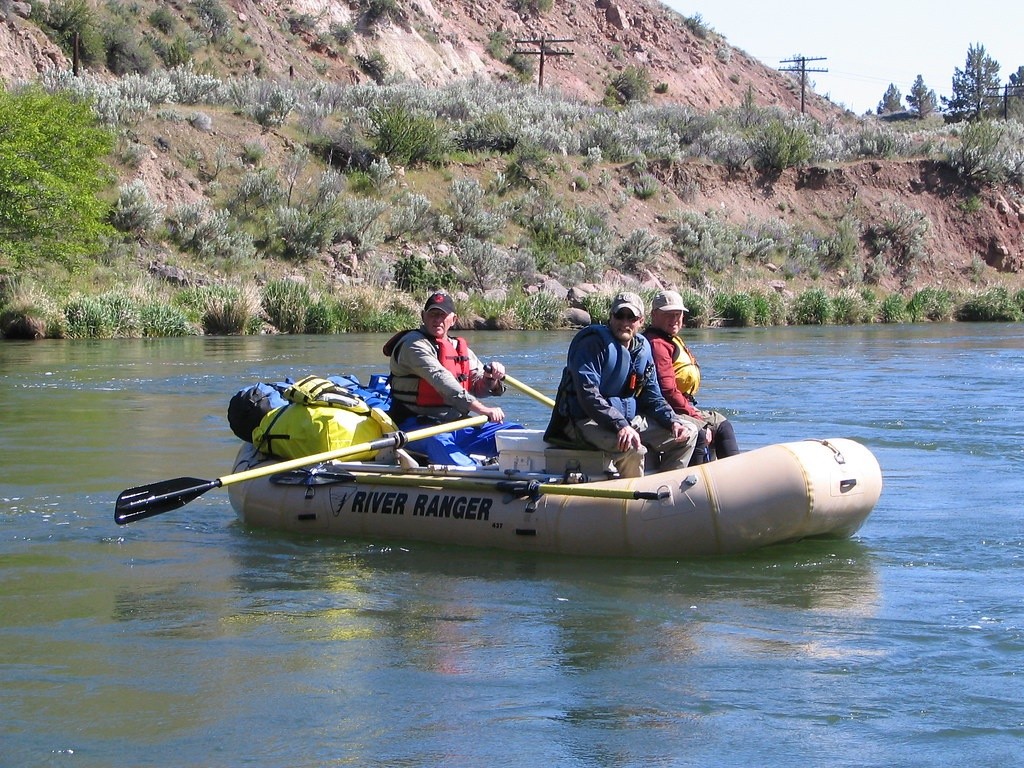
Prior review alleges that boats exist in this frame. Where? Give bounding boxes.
[227,427,886,555]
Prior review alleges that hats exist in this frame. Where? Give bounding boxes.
[652,291,690,312]
[611,292,644,316]
[424,293,455,314]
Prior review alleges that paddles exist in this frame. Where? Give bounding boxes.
[269,464,672,501]
[483,364,555,408]
[114,414,489,524]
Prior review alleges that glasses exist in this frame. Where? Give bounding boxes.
[613,312,634,319]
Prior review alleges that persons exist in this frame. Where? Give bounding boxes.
[543,292,698,479]
[641,291,740,467]
[382,293,524,466]
